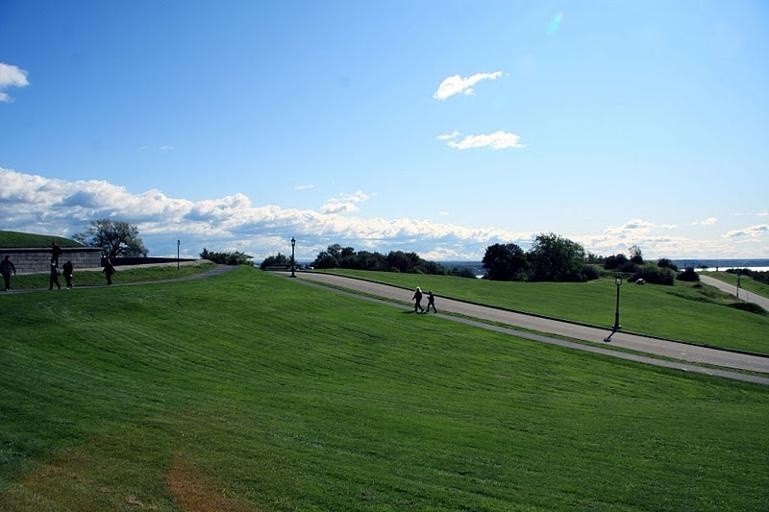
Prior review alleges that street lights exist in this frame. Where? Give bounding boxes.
[612,271,623,328]
[178,239,180,270]
[291,236,295,276]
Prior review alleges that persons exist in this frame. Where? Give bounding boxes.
[46,265,61,290]
[412,286,425,314]
[0,255,17,291]
[63,260,73,290]
[426,290,437,314]
[50,242,64,268]
[102,258,116,285]
